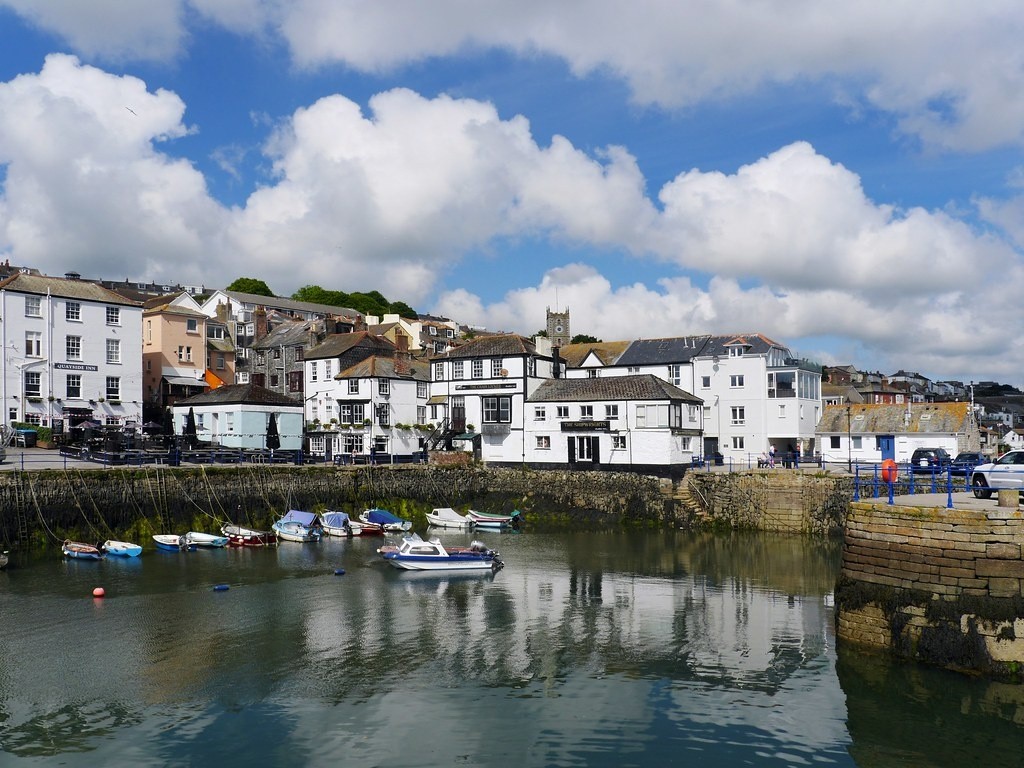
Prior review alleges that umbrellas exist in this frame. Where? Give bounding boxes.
[70,420,165,442]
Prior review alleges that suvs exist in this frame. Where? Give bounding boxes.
[971,449,1024,499]
[911,446,952,474]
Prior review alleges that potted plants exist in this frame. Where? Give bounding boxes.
[36,426,55,449]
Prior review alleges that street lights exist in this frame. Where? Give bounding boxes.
[847,406,850,472]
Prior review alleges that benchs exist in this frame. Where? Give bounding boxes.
[181,455,314,463]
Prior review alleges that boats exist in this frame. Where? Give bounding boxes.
[318,511,385,537]
[0,550,10,570]
[185,531,231,550]
[220,522,280,548]
[465,508,526,528]
[378,531,504,571]
[152,534,197,553]
[358,507,413,533]
[271,509,326,544]
[102,540,143,558]
[424,507,478,529]
[62,538,103,562]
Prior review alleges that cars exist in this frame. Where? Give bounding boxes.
[951,451,992,476]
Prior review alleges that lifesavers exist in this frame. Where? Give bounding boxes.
[883,458,898,483]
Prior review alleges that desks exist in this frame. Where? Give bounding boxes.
[182,452,278,458]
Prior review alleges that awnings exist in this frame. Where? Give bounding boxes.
[451,433,481,452]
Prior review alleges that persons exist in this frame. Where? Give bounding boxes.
[370,445,377,466]
[769,443,776,468]
[761,452,768,468]
[350,446,357,465]
